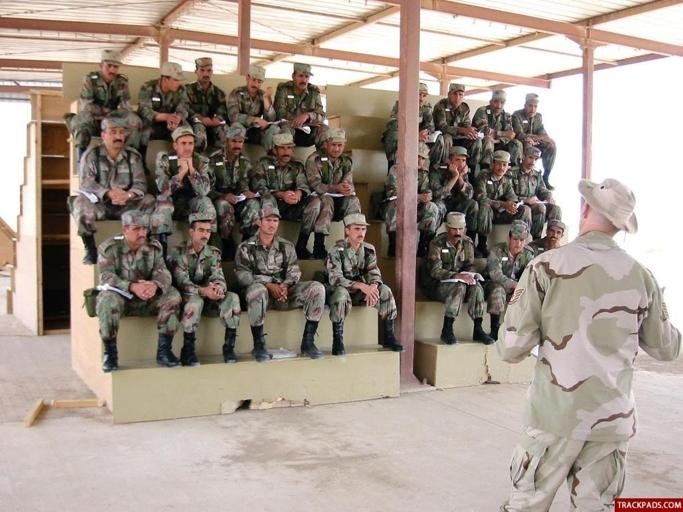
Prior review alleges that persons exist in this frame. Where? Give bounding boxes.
[210,124,261,261]
[137,63,191,176]
[70,48,141,163]
[506,147,561,242]
[73,117,155,266]
[433,84,482,180]
[167,213,241,366]
[233,208,326,362]
[150,126,217,260]
[273,63,330,149]
[184,58,230,152]
[511,93,556,191]
[313,213,405,355]
[474,150,532,259]
[251,128,321,261]
[227,65,280,155]
[382,142,438,260]
[528,220,565,258]
[419,212,496,346]
[480,220,535,343]
[304,128,361,260]
[381,83,445,176]
[431,147,483,259]
[497,178,682,511]
[471,90,524,171]
[95,210,182,372]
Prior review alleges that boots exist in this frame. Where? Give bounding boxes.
[312,233,327,260]
[418,229,434,255]
[250,324,268,362]
[542,169,554,191]
[155,234,167,265]
[155,333,180,368]
[297,231,311,260]
[331,322,346,356]
[218,237,234,262]
[222,329,238,364]
[478,235,489,258]
[471,317,495,346]
[465,231,483,260]
[488,313,501,342]
[81,234,97,264]
[440,315,459,346]
[382,320,402,352]
[241,234,255,242]
[300,321,322,358]
[101,337,119,372]
[75,147,87,169]
[139,144,151,176]
[386,230,397,258]
[179,331,200,366]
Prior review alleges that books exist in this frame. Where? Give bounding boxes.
[440,270,484,283]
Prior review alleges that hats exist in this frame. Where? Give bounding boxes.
[161,61,186,82]
[418,83,430,96]
[258,208,281,222]
[449,145,470,159]
[342,214,370,228]
[121,209,151,227]
[446,211,466,229]
[577,176,639,235]
[493,149,512,164]
[525,147,542,158]
[224,126,249,142]
[100,118,131,132]
[293,62,314,76]
[526,92,539,103]
[326,128,348,144]
[510,220,531,239]
[194,57,213,67]
[449,83,466,93]
[247,65,267,83]
[492,90,507,100]
[549,219,566,231]
[271,133,296,147]
[171,125,198,143]
[188,213,214,226]
[100,49,124,66]
[418,142,430,160]
